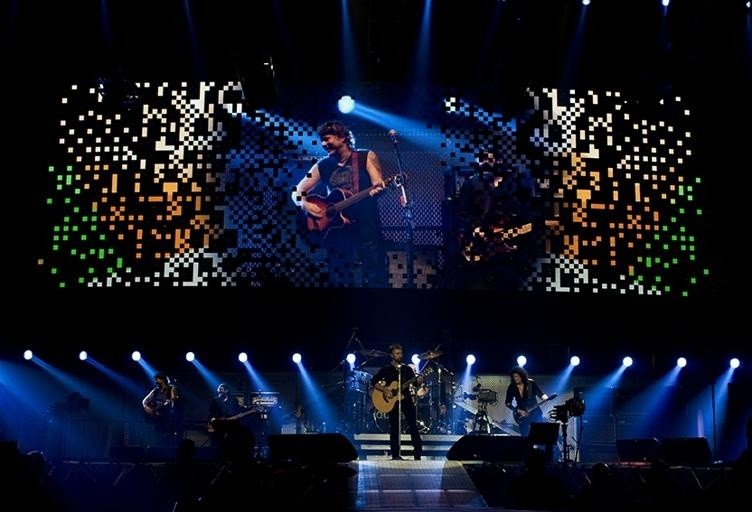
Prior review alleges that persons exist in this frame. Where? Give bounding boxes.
[505,366,548,437]
[371,344,429,461]
[451,152,547,294]
[209,384,256,424]
[142,374,183,448]
[292,122,391,289]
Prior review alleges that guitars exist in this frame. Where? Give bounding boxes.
[513,394,558,425]
[299,172,408,243]
[207,407,257,433]
[371,367,433,413]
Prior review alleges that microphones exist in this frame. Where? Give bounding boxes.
[389,129,400,146]
[397,356,403,367]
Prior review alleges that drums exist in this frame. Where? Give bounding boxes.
[349,370,373,396]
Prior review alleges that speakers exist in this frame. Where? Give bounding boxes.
[111,445,144,463]
[64,441,96,460]
[268,432,357,463]
[616,436,660,461]
[659,436,713,464]
[446,434,533,463]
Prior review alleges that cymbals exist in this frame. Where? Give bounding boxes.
[417,350,445,360]
[359,349,388,358]
